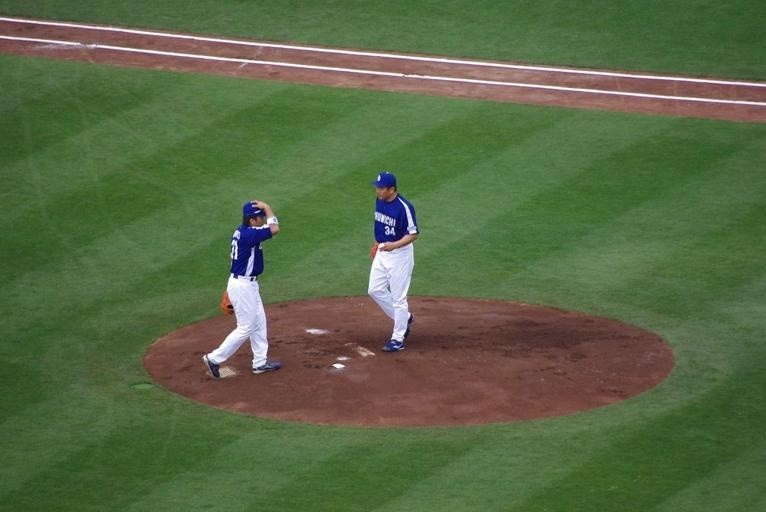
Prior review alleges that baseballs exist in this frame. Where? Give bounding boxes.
[379,243,383,248]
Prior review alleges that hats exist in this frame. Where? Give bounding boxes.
[242,200,267,217]
[370,171,397,189]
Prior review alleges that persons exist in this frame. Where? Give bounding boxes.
[367,169,421,353]
[202,198,284,378]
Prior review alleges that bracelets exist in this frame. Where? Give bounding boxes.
[265,216,279,226]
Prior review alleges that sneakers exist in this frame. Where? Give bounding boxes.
[202,354,221,379]
[381,338,405,352]
[404,311,413,340]
[251,361,280,374]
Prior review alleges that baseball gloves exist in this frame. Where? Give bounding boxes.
[222,292,234,315]
